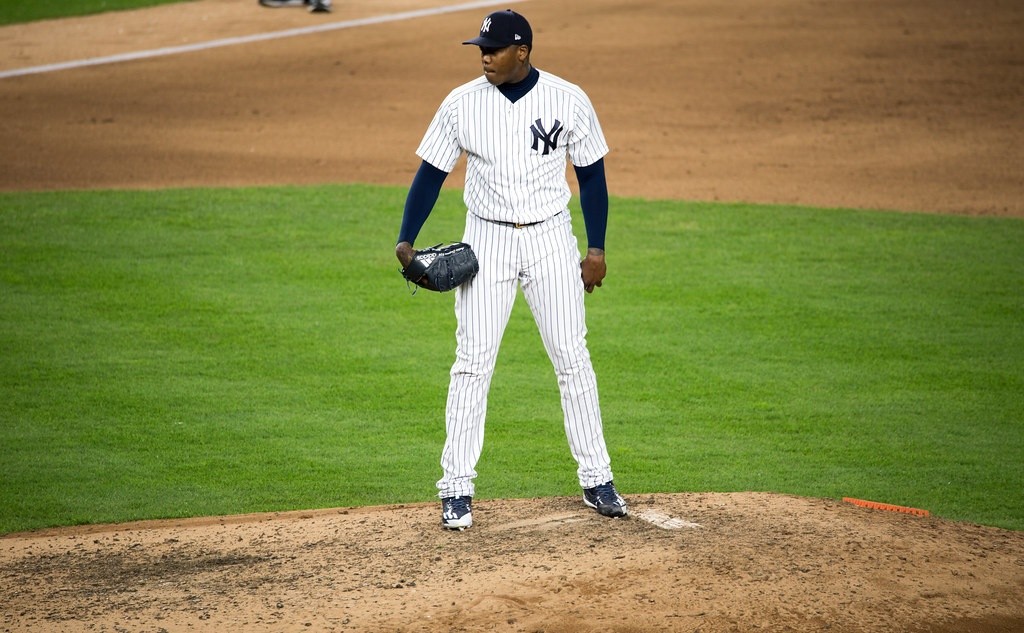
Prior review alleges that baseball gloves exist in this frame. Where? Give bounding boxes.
[395,239,481,297]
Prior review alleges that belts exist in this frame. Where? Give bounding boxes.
[473,211,562,229]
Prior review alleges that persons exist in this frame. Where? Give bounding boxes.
[258,0,331,13]
[395,9,627,531]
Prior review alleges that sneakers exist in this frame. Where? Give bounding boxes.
[583,481,627,516]
[442,496,472,529]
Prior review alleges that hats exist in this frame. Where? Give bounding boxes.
[462,9,533,48]
